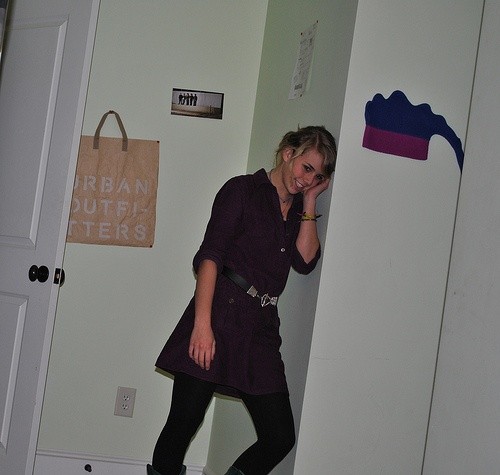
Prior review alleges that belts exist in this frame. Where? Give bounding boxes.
[222,265,278,307]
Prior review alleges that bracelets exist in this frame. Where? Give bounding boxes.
[298,212,323,222]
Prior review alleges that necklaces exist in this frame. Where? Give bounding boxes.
[269,168,291,216]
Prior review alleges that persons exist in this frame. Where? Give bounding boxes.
[145,124,338,474]
[179,93,198,106]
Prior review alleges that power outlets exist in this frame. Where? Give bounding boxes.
[113,387,137,417]
